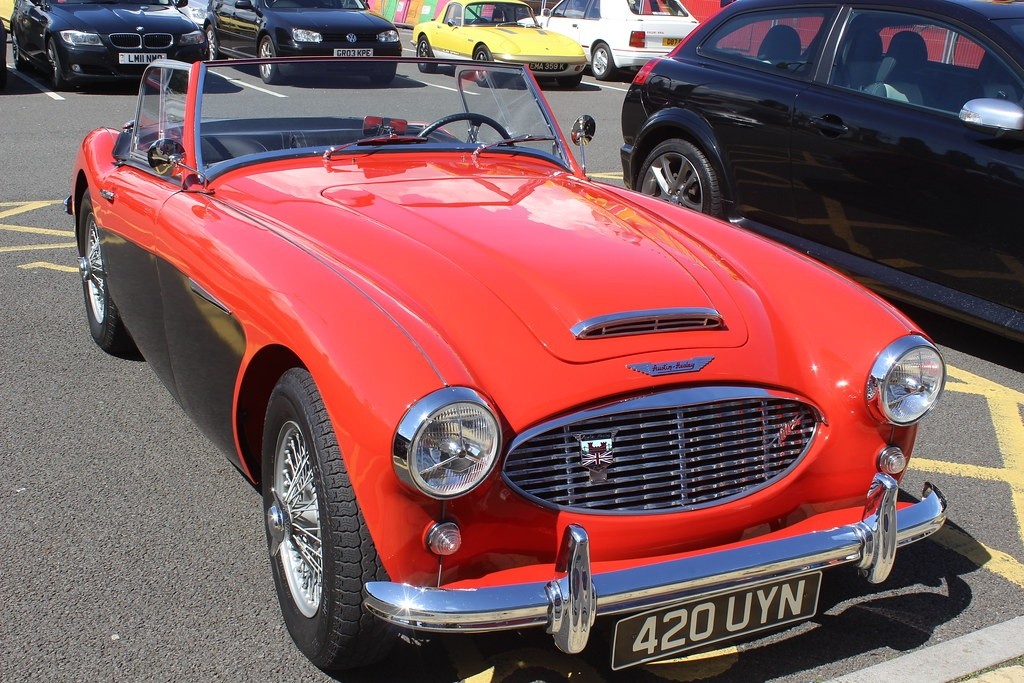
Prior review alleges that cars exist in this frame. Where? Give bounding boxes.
[203,0,402,84]
[409,0,588,88]
[64,60,947,683]
[10,0,210,92]
[516,0,700,81]
[620,0,1024,345]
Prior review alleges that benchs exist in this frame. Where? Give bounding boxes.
[143,128,367,151]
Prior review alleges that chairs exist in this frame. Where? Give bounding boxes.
[976,55,1019,104]
[757,25,802,59]
[577,0,599,18]
[201,135,268,165]
[846,29,883,90]
[863,30,929,105]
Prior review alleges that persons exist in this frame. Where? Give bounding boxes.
[873,31,944,110]
